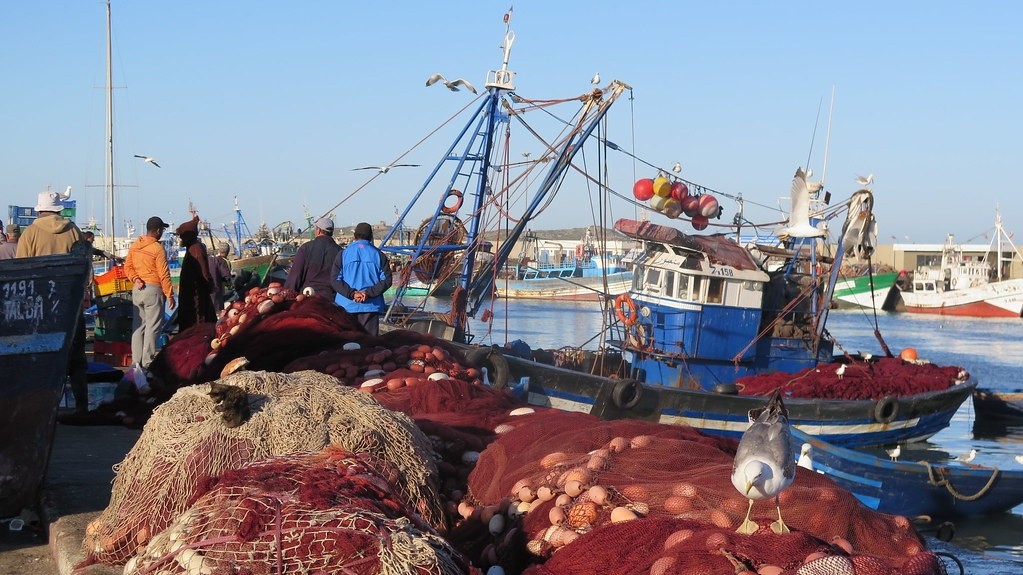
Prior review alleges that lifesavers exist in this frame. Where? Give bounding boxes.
[612,378,644,410]
[711,383,740,395]
[952,278,957,286]
[462,347,509,390]
[615,294,637,326]
[439,189,464,214]
[875,395,899,425]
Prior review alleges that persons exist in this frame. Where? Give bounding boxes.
[0,191,122,416]
[124,216,176,372]
[284,218,343,302]
[328,222,392,337]
[175,215,232,334]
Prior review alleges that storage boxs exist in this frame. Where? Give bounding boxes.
[8,200,76,236]
[59,263,135,410]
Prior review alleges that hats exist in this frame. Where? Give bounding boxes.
[0,221,4,228]
[147,217,169,228]
[218,243,230,254]
[317,218,334,233]
[6,224,20,233]
[356,223,372,237]
[35,192,64,211]
[178,216,200,243]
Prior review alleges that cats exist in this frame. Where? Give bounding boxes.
[207,380,249,428]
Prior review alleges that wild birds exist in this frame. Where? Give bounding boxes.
[953,449,977,464]
[425,72,478,96]
[863,354,873,362]
[672,161,682,177]
[589,72,601,89]
[349,164,421,174]
[884,445,900,462]
[835,364,847,379]
[855,174,874,188]
[729,384,797,537]
[59,185,72,202]
[772,166,828,240]
[134,155,161,168]
[796,442,815,471]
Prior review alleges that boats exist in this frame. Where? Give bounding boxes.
[821,268,902,311]
[971,385,1023,423]
[374,5,981,450]
[895,204,1023,322]
[788,424,1023,519]
[85,0,633,334]
[0,240,94,519]
